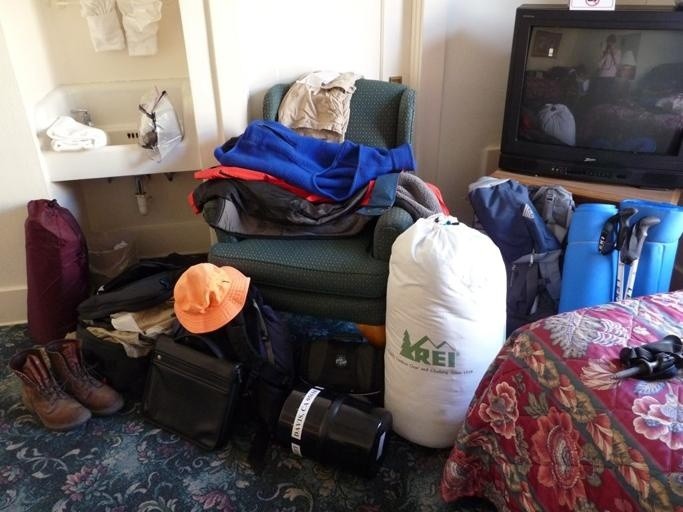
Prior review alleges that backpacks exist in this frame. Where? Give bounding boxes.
[224,281,385,411]
[467,176,576,331]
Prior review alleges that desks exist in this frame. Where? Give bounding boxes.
[483,172,680,208]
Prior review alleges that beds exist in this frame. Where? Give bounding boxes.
[479,289,683,512]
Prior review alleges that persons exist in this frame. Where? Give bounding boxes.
[596,44,618,94]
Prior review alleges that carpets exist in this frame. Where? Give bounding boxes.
[0,317,533,512]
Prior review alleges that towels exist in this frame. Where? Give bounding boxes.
[117,0,163,56]
[81,0,125,53]
[47,116,107,152]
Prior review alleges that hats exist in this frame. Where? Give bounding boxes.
[174,263,252,334]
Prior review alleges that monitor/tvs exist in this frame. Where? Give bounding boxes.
[498,4,683,190]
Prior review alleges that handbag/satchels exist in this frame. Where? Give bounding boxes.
[137,86,185,163]
[143,334,244,452]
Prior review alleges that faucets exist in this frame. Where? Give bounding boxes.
[70,109,97,127]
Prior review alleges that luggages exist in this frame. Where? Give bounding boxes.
[77,261,182,394]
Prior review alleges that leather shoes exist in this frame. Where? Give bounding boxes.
[9,339,124,431]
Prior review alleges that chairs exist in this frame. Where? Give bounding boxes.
[209,79,416,346]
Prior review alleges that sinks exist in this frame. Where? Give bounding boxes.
[98,125,142,147]
